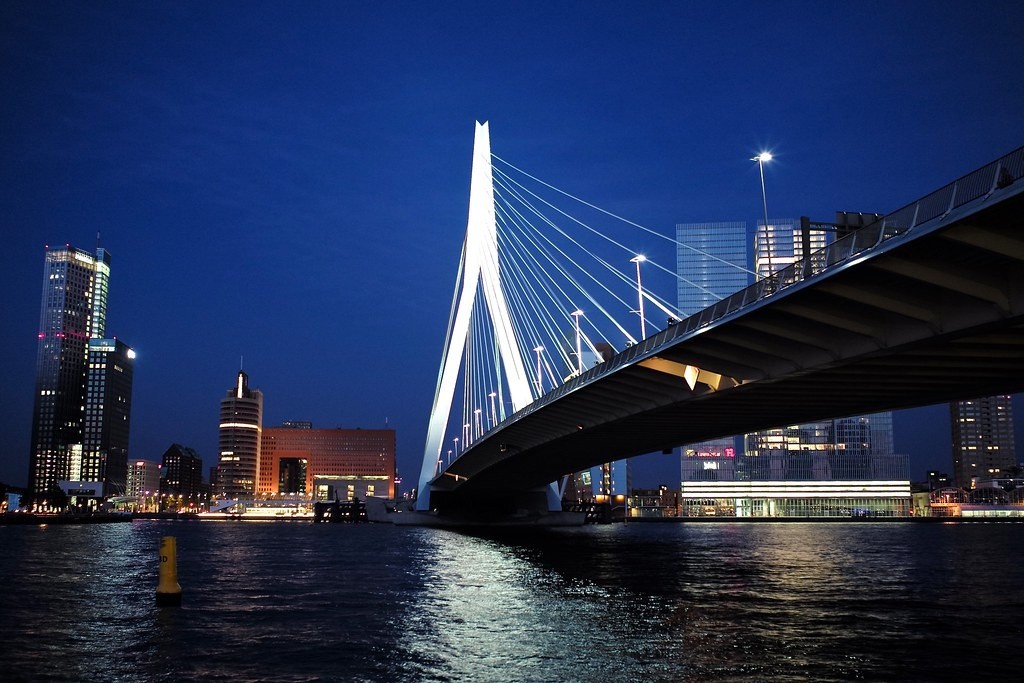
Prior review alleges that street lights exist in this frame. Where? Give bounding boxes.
[438,459,442,474]
[759,151,776,292]
[452,437,459,458]
[631,253,644,340]
[463,423,470,448]
[533,345,546,397]
[446,450,453,465]
[474,409,481,441]
[570,309,584,376]
[488,392,497,428]
